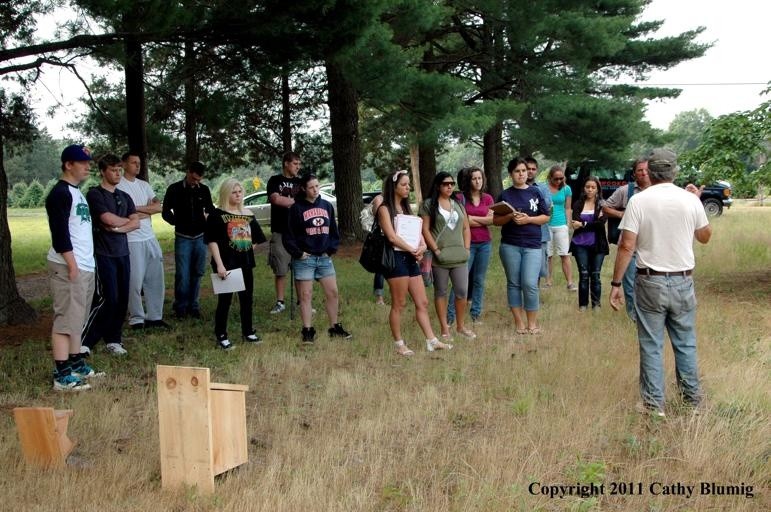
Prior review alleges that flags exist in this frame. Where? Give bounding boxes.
[252,177,260,192]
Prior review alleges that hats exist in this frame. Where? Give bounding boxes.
[647,148,678,173]
[61,145,94,162]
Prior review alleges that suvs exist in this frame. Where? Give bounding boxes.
[564,158,733,218]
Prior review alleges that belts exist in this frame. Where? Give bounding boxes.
[635,267,693,277]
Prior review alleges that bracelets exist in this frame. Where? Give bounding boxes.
[582,220,584,227]
[433,248,439,252]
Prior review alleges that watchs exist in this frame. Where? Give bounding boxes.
[611,282,622,286]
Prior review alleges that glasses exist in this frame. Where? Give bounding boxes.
[527,167,537,173]
[440,181,456,188]
[550,177,564,182]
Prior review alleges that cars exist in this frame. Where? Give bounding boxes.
[243,190,338,224]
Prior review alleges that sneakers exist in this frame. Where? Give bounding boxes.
[310,308,316,317]
[268,301,286,315]
[144,320,172,330]
[102,345,127,357]
[52,367,91,394]
[80,344,91,355]
[636,403,666,418]
[241,333,264,345]
[70,360,107,380]
[576,305,587,313]
[176,311,190,322]
[302,328,317,343]
[190,310,206,321]
[328,323,352,340]
[676,404,700,417]
[592,306,601,314]
[567,283,577,292]
[543,283,552,289]
[131,324,144,331]
[214,340,236,350]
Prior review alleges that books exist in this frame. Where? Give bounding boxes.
[209,267,247,295]
[393,213,421,252]
[488,200,519,217]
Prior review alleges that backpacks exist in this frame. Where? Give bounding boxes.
[608,183,635,244]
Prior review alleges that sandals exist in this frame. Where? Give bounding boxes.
[425,337,454,352]
[393,339,415,357]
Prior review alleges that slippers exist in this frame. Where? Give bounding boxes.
[456,329,477,340]
[529,328,540,334]
[439,334,453,343]
[514,327,526,336]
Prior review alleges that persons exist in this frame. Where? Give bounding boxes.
[607,147,711,418]
[117,151,175,332]
[376,171,453,357]
[361,176,403,306]
[86,153,141,354]
[493,156,551,334]
[603,157,668,326]
[282,173,352,344]
[417,170,478,339]
[524,155,551,289]
[267,153,306,314]
[163,161,214,322]
[540,165,576,292]
[205,178,266,350]
[447,166,494,328]
[45,145,105,392]
[568,177,609,313]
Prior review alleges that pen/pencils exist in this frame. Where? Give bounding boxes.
[222,272,231,280]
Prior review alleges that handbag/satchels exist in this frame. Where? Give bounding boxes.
[358,202,397,276]
[421,199,454,287]
